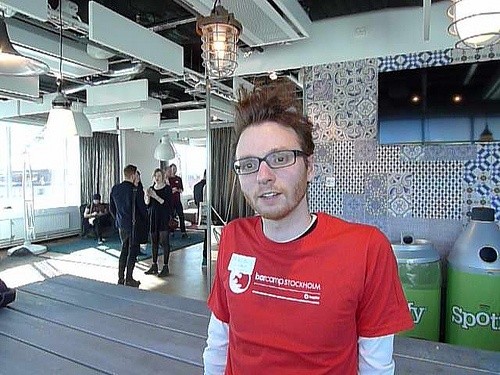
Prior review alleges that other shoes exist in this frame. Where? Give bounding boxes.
[119,279,125,285]
[127,280,140,285]
[182,233,191,238]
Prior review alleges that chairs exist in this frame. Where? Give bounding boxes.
[183,200,199,224]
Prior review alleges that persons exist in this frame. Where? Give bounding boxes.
[109,165,142,285]
[134,171,150,263]
[84,193,108,245]
[144,169,173,277]
[201,79,415,375]
[162,164,192,240]
[194,169,206,212]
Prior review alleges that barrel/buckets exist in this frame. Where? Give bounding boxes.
[445,207,500,351]
[391,231,441,341]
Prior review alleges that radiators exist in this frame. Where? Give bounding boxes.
[35,213,70,233]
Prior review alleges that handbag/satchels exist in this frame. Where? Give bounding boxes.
[132,225,148,244]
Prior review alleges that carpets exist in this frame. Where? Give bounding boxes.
[43,231,204,261]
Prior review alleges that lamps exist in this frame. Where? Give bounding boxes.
[45,0,77,134]
[479,113,492,143]
[154,136,175,161]
[0,0,50,76]
[446,1,500,48]
[69,98,93,137]
[196,0,242,79]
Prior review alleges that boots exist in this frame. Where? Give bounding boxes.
[144,264,158,274]
[158,266,169,276]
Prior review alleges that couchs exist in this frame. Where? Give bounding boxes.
[80,202,114,236]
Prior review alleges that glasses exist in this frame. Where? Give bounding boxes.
[233,150,309,175]
